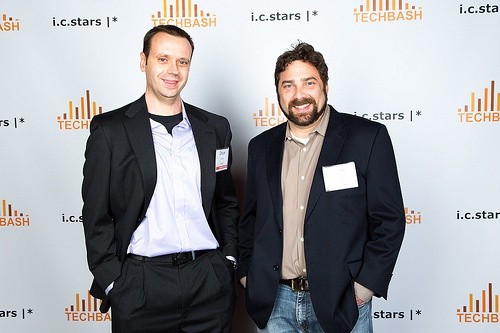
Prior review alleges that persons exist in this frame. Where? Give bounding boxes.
[82,24,240,333]
[236,41,407,333]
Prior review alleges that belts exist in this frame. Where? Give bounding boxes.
[279,276,311,292]
[125,248,215,266]
